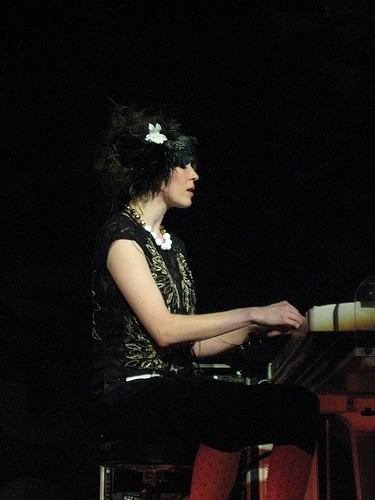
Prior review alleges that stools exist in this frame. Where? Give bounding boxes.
[99,463,190,500]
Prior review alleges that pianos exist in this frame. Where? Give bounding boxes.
[263,298,374,384]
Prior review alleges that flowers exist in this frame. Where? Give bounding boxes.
[145,123,168,145]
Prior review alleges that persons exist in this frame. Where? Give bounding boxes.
[91,106,320,500]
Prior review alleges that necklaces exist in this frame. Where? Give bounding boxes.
[124,203,173,250]
